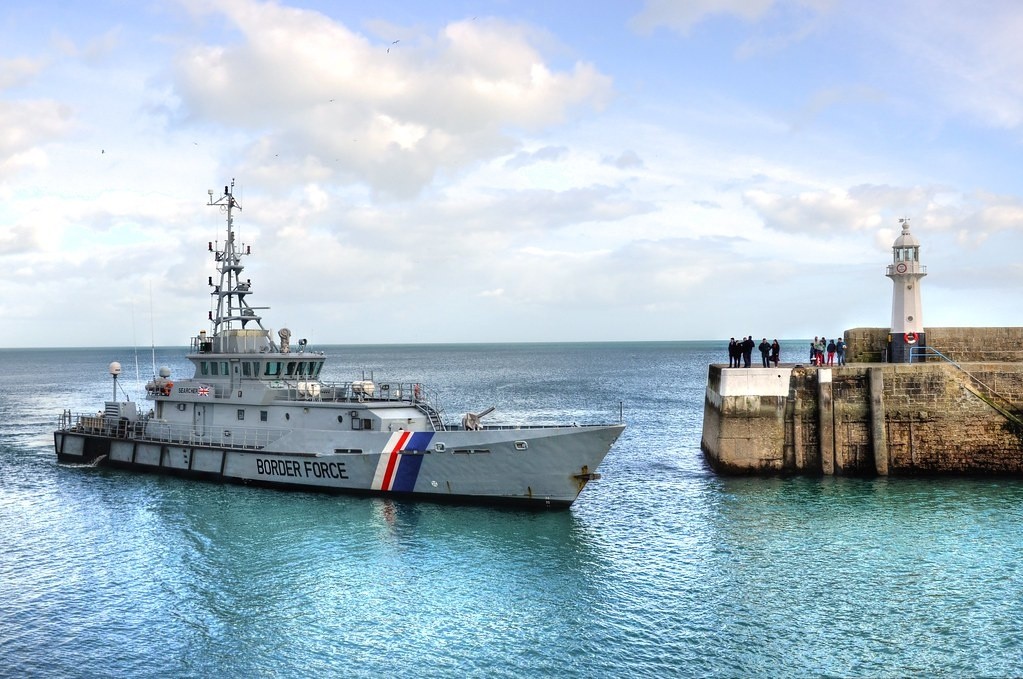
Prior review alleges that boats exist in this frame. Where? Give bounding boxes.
[52,177,625,511]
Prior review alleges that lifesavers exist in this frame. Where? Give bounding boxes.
[164,382,174,396]
[904,331,919,345]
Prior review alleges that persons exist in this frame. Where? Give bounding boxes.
[772,340,780,367]
[728,338,743,368]
[820,337,826,364]
[836,337,848,366]
[810,335,825,367]
[741,336,755,368]
[758,338,772,368]
[827,340,837,367]
[414,383,419,399]
